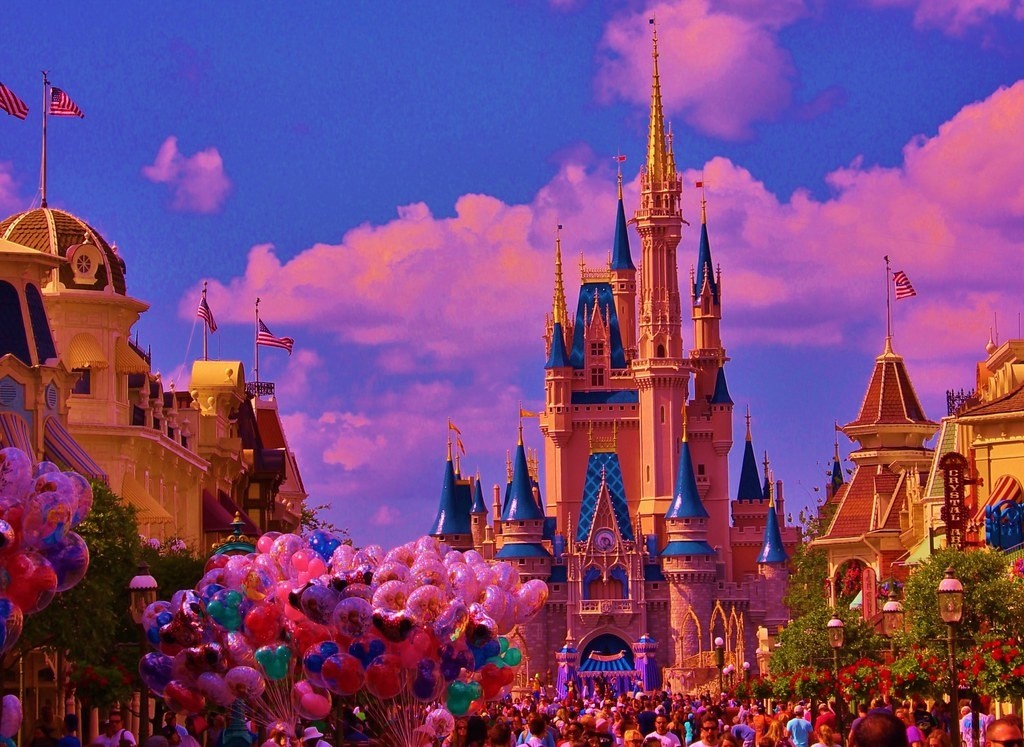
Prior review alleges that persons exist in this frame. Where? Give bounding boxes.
[31,711,200,747]
[443,672,1024,747]
[206,712,385,747]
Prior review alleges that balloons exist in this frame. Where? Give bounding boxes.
[0,449,94,734]
[139,530,549,738]
[0,694,22,739]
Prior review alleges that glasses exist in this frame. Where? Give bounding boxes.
[645,736,657,742]
[567,729,579,734]
[109,720,122,725]
[990,739,1024,747]
[165,716,174,723]
[655,721,666,724]
[702,726,718,731]
[166,729,174,739]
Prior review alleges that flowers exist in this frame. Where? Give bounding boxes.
[71,655,144,711]
[724,640,1024,706]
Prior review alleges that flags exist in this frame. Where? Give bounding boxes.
[258,319,294,357]
[0,83,30,120]
[836,425,856,444]
[49,85,85,119]
[197,302,217,334]
[892,270,916,300]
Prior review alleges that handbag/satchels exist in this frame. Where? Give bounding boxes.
[118,729,130,747]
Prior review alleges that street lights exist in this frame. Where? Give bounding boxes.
[827,610,845,747]
[129,561,160,747]
[936,562,964,747]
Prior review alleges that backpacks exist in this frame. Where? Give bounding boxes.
[551,718,560,728]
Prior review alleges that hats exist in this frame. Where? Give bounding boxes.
[300,726,324,742]
[794,705,804,714]
[106,719,110,724]
[757,706,764,712]
